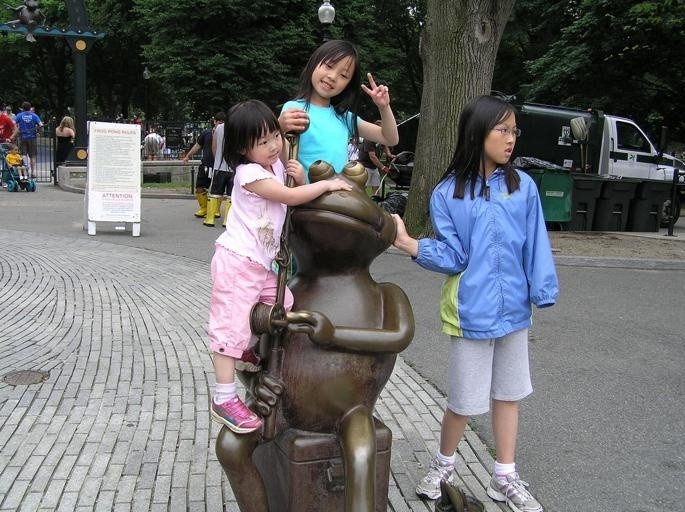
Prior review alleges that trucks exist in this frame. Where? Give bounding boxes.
[387,101,685,229]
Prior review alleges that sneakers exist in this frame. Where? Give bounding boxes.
[209,394,264,435]
[20,179,29,185]
[415,457,455,500]
[488,470,544,512]
[233,349,260,373]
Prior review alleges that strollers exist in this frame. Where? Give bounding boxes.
[1,140,38,193]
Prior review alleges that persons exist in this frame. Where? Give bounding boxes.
[277,39,399,185]
[207,98,353,435]
[182,111,226,218]
[358,121,398,196]
[203,123,235,226]
[348,139,360,161]
[1,102,171,187]
[390,93,559,512]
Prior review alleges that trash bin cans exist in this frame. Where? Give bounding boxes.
[512,157,673,232]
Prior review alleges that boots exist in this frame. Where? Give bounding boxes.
[202,196,218,227]
[194,190,209,218]
[221,196,233,227]
[214,193,222,219]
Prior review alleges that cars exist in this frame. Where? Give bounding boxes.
[659,150,685,171]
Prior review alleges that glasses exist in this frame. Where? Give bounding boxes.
[491,126,522,139]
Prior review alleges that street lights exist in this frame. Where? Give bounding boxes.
[316,0,336,42]
[142,64,153,135]
